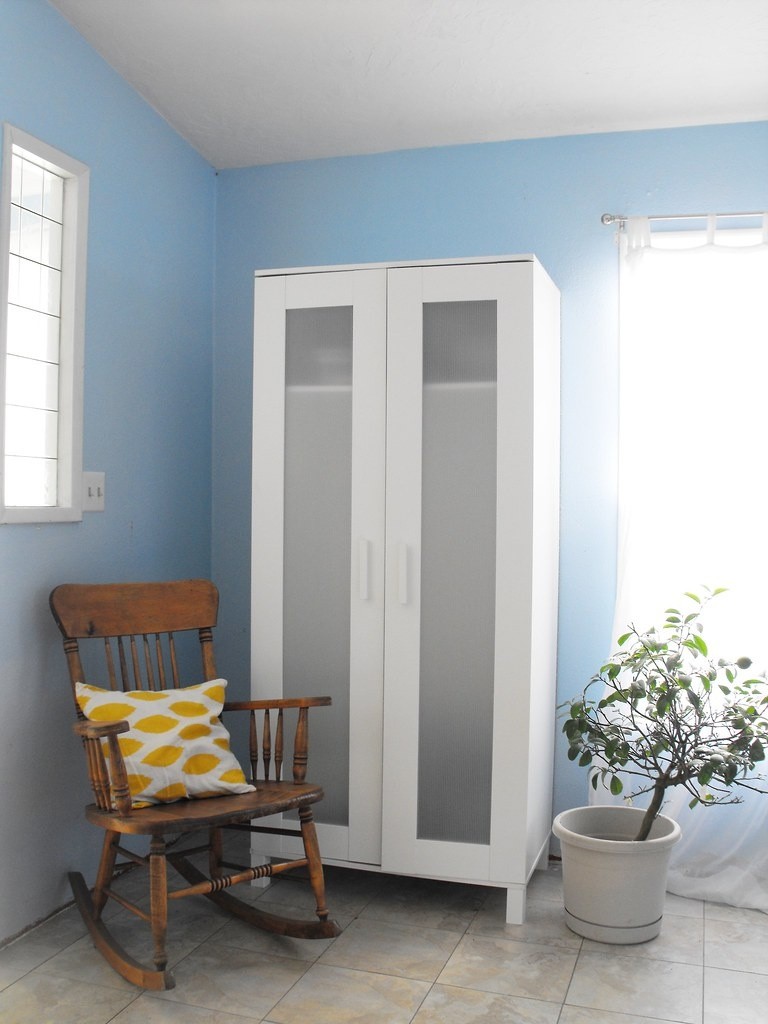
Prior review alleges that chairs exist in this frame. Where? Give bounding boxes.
[47,581,342,993]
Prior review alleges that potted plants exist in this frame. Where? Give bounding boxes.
[551,583,768,945]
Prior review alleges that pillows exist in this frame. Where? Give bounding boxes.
[73,678,257,809]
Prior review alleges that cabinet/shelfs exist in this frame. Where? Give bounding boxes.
[252,253,562,926]
